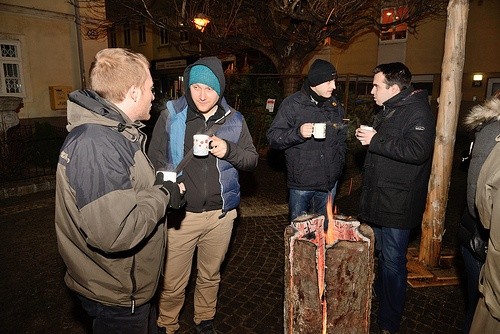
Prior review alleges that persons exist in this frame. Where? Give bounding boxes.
[148,57,260,334]
[353,62,433,334]
[55,48,187,334]
[266,58,347,234]
[459,93,500,333]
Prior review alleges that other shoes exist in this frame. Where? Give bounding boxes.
[157,327,178,334]
[192,320,216,334]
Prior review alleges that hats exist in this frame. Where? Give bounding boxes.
[188,65,221,96]
[307,59,337,87]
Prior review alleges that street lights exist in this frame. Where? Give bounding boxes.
[191,12,211,60]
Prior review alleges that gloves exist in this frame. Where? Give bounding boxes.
[152,172,186,210]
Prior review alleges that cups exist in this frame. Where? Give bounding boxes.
[355,127,373,145]
[193,135,215,157]
[311,122,326,139]
[156,170,177,182]
